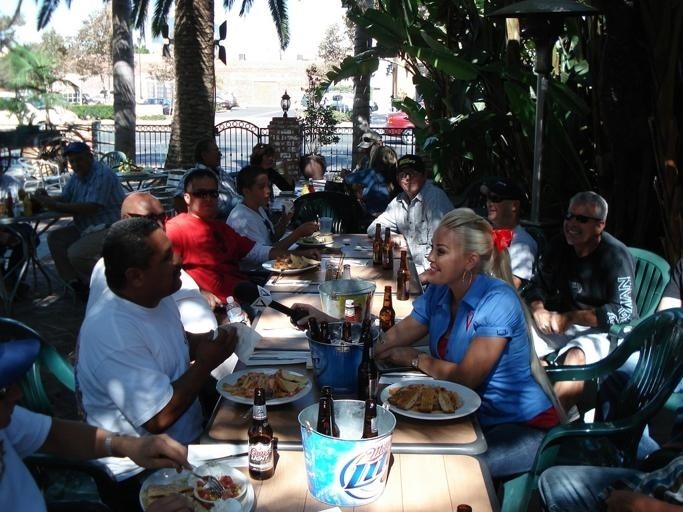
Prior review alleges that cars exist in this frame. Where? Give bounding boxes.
[301,92,378,113]
[143,97,179,115]
[382,101,426,146]
[215,95,232,112]
[0,92,62,131]
[37,103,78,123]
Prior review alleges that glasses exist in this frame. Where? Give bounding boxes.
[127,212,165,220]
[188,189,219,199]
[564,212,603,223]
[263,219,276,242]
[489,195,518,202]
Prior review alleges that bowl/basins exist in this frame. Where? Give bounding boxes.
[193,466,246,503]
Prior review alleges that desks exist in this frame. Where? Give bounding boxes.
[198,233,494,509]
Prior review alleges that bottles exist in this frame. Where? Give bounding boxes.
[299,176,315,196]
[371,223,393,269]
[379,286,394,330]
[363,400,378,440]
[341,265,351,293]
[358,332,380,404]
[245,388,275,481]
[223,295,246,324]
[317,384,340,438]
[396,250,409,299]
[457,502,471,512]
[309,316,370,341]
[0,189,42,216]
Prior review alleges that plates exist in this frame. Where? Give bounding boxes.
[138,461,255,512]
[260,257,320,274]
[292,237,335,248]
[379,378,482,420]
[215,368,311,406]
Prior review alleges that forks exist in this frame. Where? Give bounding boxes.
[180,466,224,495]
[271,266,287,284]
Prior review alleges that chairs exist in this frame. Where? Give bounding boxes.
[1,148,400,234]
[1,317,132,512]
[495,219,682,512]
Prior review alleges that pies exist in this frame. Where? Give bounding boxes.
[398,390,460,414]
[273,367,302,393]
[143,482,192,508]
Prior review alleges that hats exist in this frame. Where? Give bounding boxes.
[61,141,89,156]
[397,154,425,173]
[490,179,519,195]
[0,337,41,390]
[356,131,382,149]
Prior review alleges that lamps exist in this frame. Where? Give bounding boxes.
[279,89,291,118]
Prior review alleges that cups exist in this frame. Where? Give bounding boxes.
[318,217,333,235]
[325,258,340,281]
